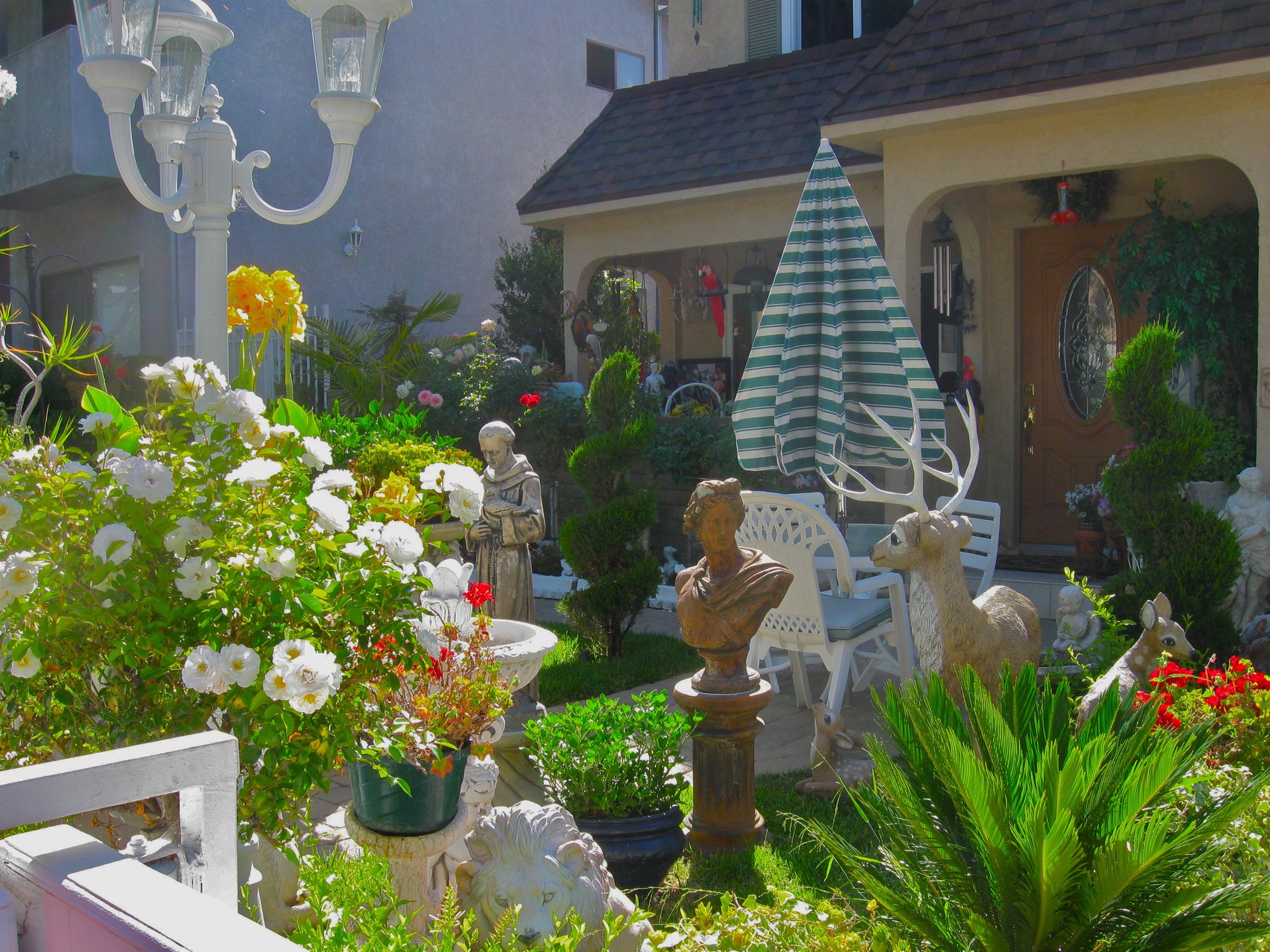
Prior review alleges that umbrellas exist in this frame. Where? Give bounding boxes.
[731,138,949,540]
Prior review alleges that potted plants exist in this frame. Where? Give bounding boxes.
[522,685,706,902]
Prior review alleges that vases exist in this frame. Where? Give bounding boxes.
[1104,511,1125,539]
[349,737,476,832]
[1071,528,1105,561]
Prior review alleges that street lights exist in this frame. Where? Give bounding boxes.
[73,0,420,392]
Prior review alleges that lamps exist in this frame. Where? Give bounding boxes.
[343,216,365,259]
[1049,182,1079,226]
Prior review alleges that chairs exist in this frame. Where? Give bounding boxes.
[935,493,1002,595]
[732,486,917,723]
[747,488,830,693]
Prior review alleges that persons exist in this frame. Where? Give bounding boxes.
[645,362,666,406]
[1217,467,1270,632]
[1052,585,1089,664]
[465,421,546,707]
[675,478,794,693]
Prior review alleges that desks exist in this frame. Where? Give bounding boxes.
[776,516,905,700]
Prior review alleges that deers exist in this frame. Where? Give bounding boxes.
[820,386,1043,709]
[1076,591,1202,735]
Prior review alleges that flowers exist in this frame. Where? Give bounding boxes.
[1064,442,1134,530]
[0,258,581,832]
